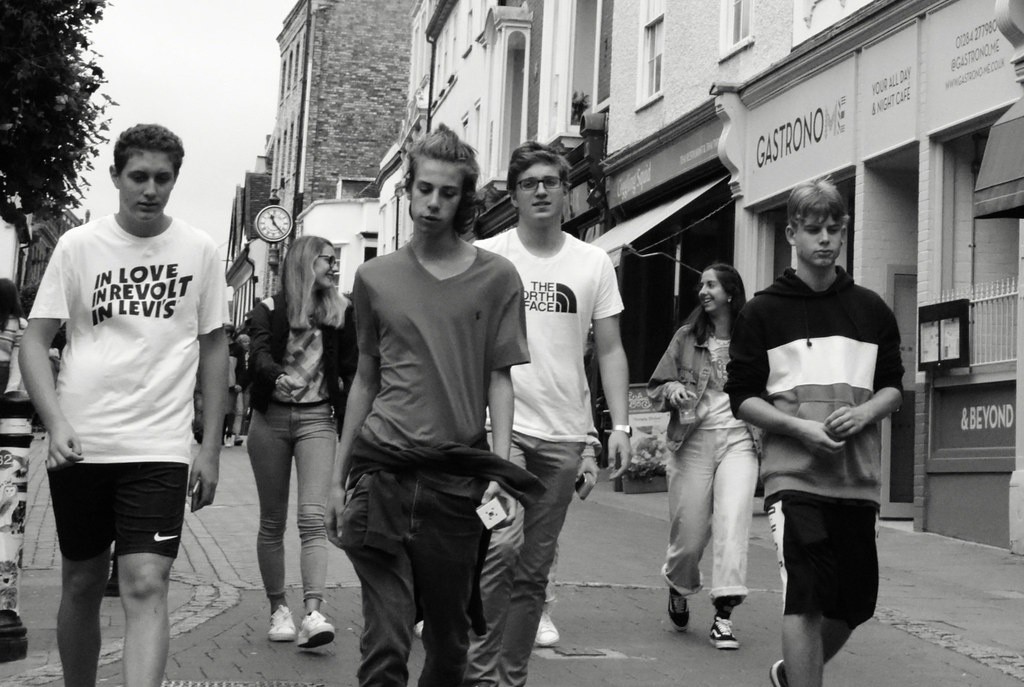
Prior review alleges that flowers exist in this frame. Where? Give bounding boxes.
[620,449,666,481]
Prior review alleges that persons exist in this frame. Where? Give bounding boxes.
[246,236,360,649]
[193,326,253,448]
[18,124,234,687]
[723,177,906,687]
[413,353,602,646]
[466,140,632,687]
[645,263,767,650]
[0,278,30,418]
[323,124,530,687]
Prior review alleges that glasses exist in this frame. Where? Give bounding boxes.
[516,176,563,191]
[318,255,336,268]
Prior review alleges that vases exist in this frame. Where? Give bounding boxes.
[621,475,668,493]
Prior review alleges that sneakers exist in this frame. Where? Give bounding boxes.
[668,592,689,632]
[535,613,560,647]
[769,659,789,687]
[297,610,335,648]
[709,616,739,650]
[267,604,296,641]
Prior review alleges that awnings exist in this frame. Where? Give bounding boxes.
[587,173,731,269]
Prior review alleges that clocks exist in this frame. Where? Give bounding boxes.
[254,204,294,242]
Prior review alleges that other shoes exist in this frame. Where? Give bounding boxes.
[225,437,231,447]
[413,620,424,639]
[234,436,243,446]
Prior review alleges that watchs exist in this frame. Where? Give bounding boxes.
[613,424,633,437]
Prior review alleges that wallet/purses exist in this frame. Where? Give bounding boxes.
[576,473,597,499]
